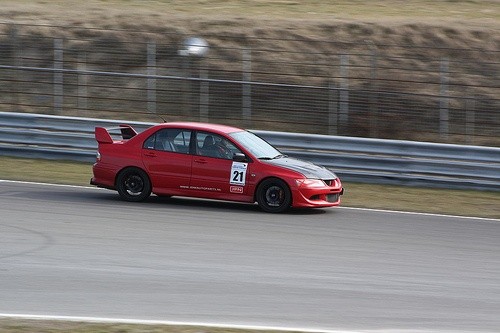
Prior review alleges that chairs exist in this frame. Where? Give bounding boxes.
[203,136,226,158]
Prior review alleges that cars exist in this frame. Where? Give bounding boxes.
[89,121,344,212]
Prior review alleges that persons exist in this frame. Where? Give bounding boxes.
[212,135,233,159]
